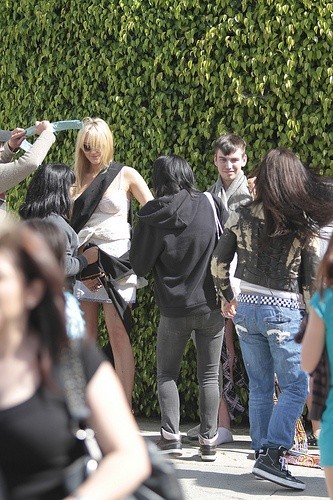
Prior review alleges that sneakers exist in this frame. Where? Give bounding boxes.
[253,446,269,480]
[196,432,219,461]
[155,428,183,458]
[252,445,306,491]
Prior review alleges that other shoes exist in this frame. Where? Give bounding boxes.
[306,432,322,446]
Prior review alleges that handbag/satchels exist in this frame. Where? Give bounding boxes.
[64,438,185,500]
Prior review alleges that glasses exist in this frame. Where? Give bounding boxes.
[84,144,91,152]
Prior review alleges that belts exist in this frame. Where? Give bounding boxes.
[237,293,300,309]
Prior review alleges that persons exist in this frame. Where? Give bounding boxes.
[71,118,155,406]
[17,163,98,295]
[211,149,322,490]
[307,178,333,421]
[130,154,231,460]
[188,133,257,446]
[22,220,86,340]
[0,121,55,195]
[0,220,152,500]
[300,230,333,500]
